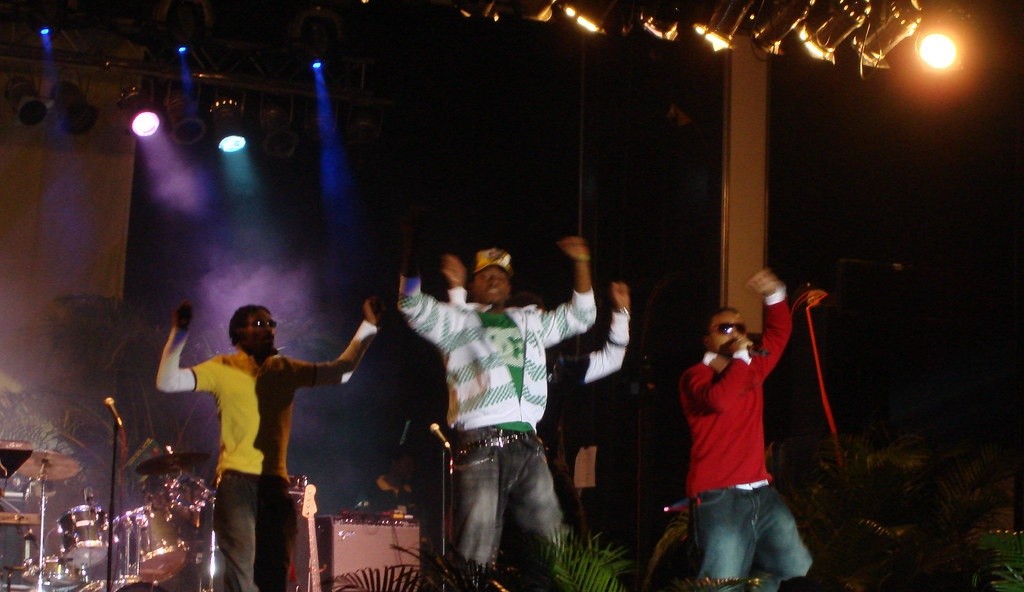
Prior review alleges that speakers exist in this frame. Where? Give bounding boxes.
[316,514,424,592]
[207,490,314,592]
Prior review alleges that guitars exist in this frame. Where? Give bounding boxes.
[295,475,323,592]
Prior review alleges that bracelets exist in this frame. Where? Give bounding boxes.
[574,255,591,263]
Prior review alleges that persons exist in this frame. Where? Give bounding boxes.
[397,222,630,569]
[680,269,815,592]
[156,297,385,592]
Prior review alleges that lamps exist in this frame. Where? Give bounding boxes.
[209,87,247,153]
[459,0,923,80]
[4,62,47,126]
[257,89,298,159]
[302,98,340,160]
[161,81,206,144]
[346,105,384,154]
[50,70,98,134]
[115,76,160,138]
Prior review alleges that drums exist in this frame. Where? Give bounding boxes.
[142,464,217,521]
[21,556,89,590]
[107,503,192,583]
[54,504,111,569]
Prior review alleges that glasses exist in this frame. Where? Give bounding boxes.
[244,320,279,329]
[717,322,747,335]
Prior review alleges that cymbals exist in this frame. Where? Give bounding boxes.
[134,449,214,477]
[14,448,83,482]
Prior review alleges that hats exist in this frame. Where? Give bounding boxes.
[467,247,515,277]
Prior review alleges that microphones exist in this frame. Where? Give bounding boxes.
[431,423,451,448]
[104,397,123,428]
[747,344,771,358]
